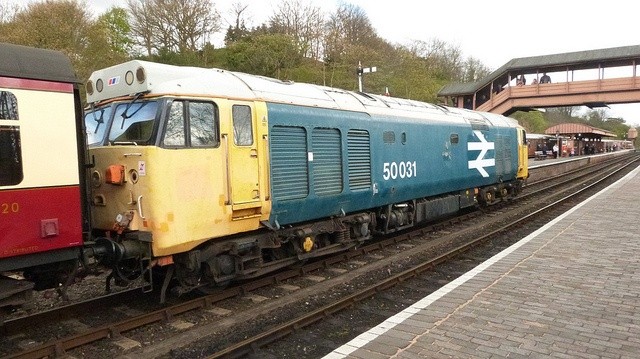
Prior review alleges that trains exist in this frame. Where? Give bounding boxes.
[0,39,94,290]
[82,60,530,292]
